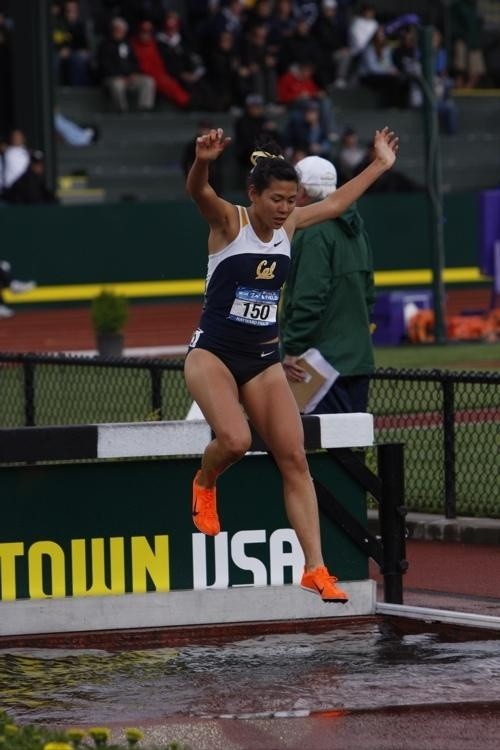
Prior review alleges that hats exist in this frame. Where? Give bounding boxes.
[294,155,338,198]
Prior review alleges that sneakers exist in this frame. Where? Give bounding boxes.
[300,566,349,605]
[190,468,221,538]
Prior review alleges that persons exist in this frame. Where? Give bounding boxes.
[1,101,96,200]
[1,262,38,318]
[171,121,400,603]
[275,155,378,413]
[49,1,500,181]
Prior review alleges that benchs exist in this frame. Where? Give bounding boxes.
[46,76,500,207]
